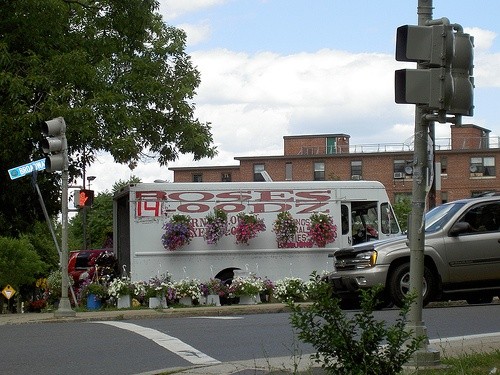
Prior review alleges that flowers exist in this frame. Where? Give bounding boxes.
[22,252,326,308]
[232,210,266,246]
[271,210,300,247]
[367,224,378,238]
[307,210,339,248]
[202,209,231,245]
[160,214,196,251]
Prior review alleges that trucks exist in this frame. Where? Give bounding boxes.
[113,171,402,305]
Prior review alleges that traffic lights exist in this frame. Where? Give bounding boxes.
[75,189,95,208]
[41,117,68,173]
[394,16,454,110]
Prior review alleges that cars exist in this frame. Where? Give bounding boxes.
[328,195,500,308]
[68,249,113,304]
